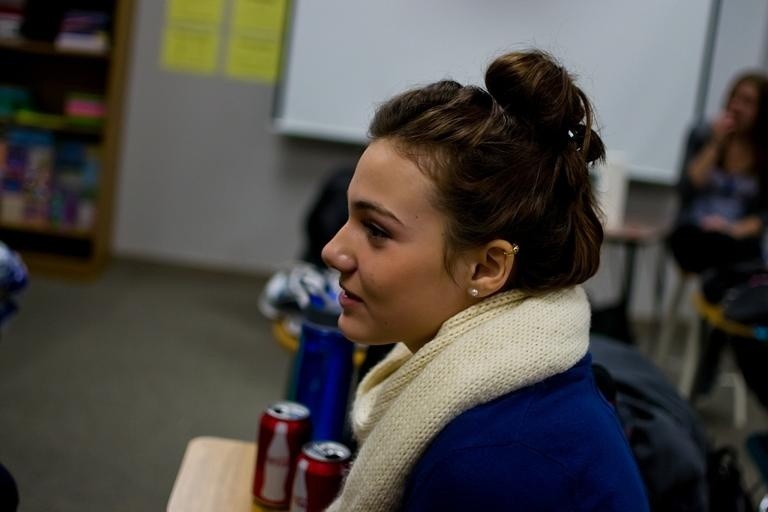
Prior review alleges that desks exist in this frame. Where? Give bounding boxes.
[591,224,674,346]
[163,436,295,512]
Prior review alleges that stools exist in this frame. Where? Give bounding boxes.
[287,317,355,444]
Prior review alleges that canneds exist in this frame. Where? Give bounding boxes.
[253,401,311,508]
[289,440,352,512]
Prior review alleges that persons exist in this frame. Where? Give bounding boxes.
[664,72,768,413]
[322,51,652,511]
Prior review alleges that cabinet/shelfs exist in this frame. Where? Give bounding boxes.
[0,0,138,277]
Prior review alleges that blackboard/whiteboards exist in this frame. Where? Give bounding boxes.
[268,0,721,190]
